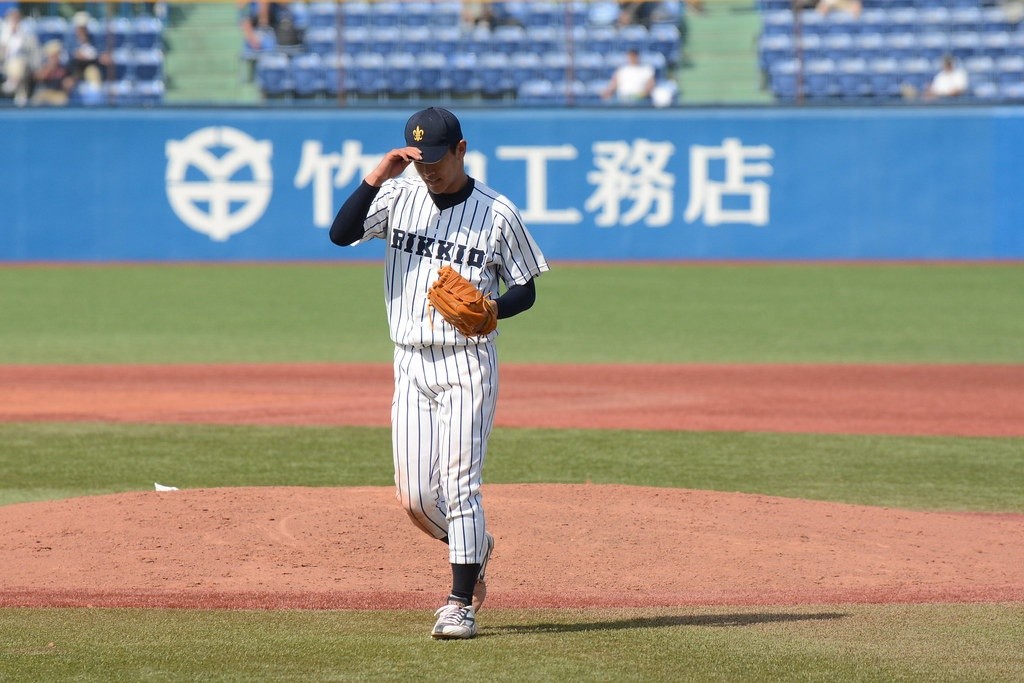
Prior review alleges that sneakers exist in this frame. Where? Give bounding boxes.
[473,530,494,614]
[431,594,476,638]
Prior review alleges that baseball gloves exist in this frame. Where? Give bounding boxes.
[425,264,497,338]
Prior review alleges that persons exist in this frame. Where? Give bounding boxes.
[0,10,108,107]
[814,0,861,16]
[462,0,495,33]
[600,49,655,100]
[242,0,292,48]
[617,0,662,32]
[923,53,969,98]
[329,106,550,640]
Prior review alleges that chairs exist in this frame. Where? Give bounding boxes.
[756,0,1024,106]
[0,0,166,104]
[240,0,684,107]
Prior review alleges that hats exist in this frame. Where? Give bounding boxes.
[401,106,461,164]
